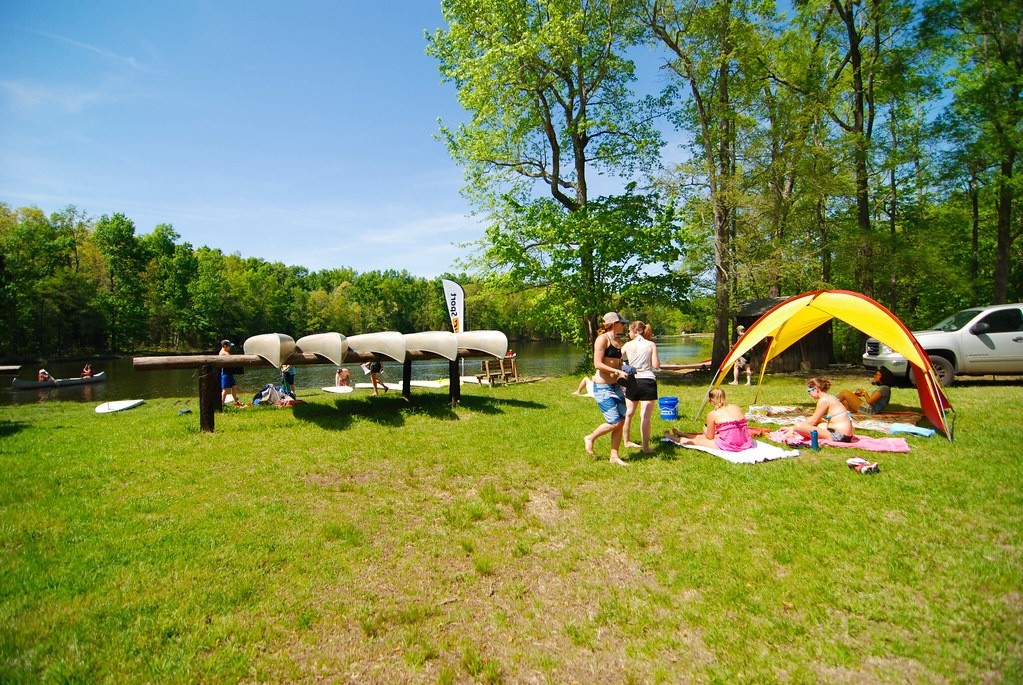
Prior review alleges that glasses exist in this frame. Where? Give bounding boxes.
[807,387,814,393]
[628,328,634,334]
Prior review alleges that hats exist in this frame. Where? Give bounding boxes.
[220,340,234,346]
[734,325,745,331]
[602,312,629,324]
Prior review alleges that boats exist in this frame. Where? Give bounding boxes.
[455,330,508,361]
[406,330,458,360]
[293,332,349,369]
[12,371,108,389]
[243,333,295,369]
[348,332,407,366]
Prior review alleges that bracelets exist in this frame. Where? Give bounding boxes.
[615,369,619,376]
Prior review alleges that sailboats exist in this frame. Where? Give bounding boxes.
[441,278,499,386]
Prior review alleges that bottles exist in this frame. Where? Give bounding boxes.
[380,369,389,379]
[812,430,818,451]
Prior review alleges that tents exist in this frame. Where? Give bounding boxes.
[695,289,958,442]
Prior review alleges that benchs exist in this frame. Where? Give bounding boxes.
[476,356,518,390]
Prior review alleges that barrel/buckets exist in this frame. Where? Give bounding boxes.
[658,397,679,420]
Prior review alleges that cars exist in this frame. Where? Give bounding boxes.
[862,302,1023,388]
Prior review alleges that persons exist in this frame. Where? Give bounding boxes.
[835,369,894,414]
[38,368,54,381]
[728,326,751,386]
[572,312,661,467]
[362,361,388,396]
[335,368,352,387]
[663,387,754,453]
[281,364,296,397]
[779,376,853,442]
[83,365,93,378]
[219,339,242,408]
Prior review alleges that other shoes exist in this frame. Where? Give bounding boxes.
[743,383,750,386]
[729,380,738,385]
[846,456,879,474]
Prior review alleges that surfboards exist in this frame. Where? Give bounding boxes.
[95,400,145,414]
[322,376,496,394]
